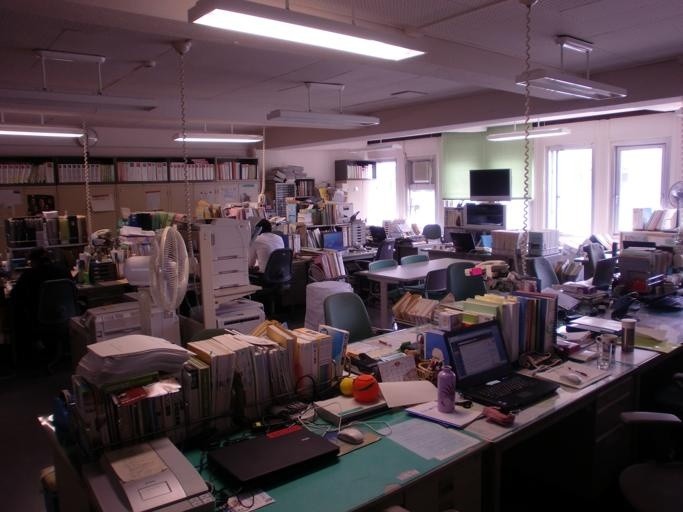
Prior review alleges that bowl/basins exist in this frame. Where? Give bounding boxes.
[42,211,59,218]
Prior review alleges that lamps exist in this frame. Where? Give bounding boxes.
[0,48,158,112]
[515,35,629,100]
[187,1,428,63]
[266,81,381,128]
[172,120,265,144]
[0,109,84,138]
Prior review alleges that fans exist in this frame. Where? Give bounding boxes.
[668,180,683,227]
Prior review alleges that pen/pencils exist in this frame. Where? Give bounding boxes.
[379,340,392,346]
[501,410,521,414]
[568,367,587,376]
[427,360,443,371]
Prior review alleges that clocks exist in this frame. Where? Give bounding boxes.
[78,127,98,147]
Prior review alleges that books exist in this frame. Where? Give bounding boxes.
[305,226,343,250]
[268,164,288,183]
[403,398,486,429]
[312,251,347,283]
[57,159,216,183]
[276,184,295,218]
[423,276,558,369]
[313,393,388,427]
[296,180,314,197]
[339,226,353,249]
[565,313,623,341]
[70,324,350,451]
[109,208,188,279]
[216,159,256,180]
[344,341,418,382]
[5,214,89,248]
[390,293,439,324]
[488,228,525,256]
[0,162,55,185]
[618,204,681,296]
[317,185,350,226]
[346,163,374,180]
[273,223,301,235]
[528,229,559,257]
[555,232,611,283]
[287,233,301,259]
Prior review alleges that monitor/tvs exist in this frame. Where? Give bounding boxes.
[466,203,507,231]
[468,168,513,201]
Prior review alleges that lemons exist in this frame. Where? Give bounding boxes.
[340,377,355,395]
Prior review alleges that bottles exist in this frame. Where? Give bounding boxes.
[438,365,456,413]
[596,337,613,370]
[620,318,637,351]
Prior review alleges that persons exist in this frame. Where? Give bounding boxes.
[10,245,77,377]
[248,218,285,320]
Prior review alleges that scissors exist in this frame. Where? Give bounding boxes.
[434,400,472,408]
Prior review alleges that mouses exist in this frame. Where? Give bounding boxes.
[337,428,363,446]
[561,371,582,385]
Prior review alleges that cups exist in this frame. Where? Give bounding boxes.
[601,333,618,366]
[655,286,663,295]
[664,283,673,295]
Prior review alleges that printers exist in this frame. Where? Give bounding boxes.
[82,436,218,512]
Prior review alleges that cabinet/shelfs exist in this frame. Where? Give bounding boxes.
[0,156,260,250]
[336,159,376,180]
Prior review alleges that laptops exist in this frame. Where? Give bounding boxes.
[585,256,619,288]
[205,423,340,493]
[449,232,486,252]
[443,318,561,416]
[322,230,347,253]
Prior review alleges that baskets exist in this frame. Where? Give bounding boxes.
[416,361,441,387]
